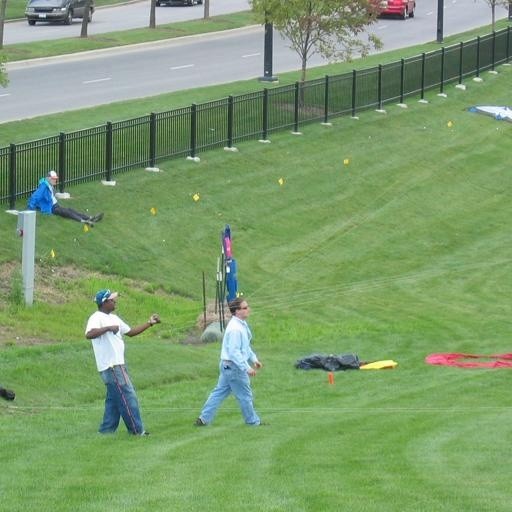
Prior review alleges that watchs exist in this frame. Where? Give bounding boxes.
[148,320,153,327]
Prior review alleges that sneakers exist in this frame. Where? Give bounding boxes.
[196,417,207,426]
[90,212,104,222]
[84,220,93,227]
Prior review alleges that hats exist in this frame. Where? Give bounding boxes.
[46,170,58,179]
[95,289,117,306]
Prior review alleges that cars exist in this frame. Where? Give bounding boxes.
[26,0,95,25]
[364,0,416,20]
[155,0,202,7]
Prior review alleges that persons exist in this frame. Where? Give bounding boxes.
[85,289,160,436]
[28,170,104,227]
[195,298,262,426]
